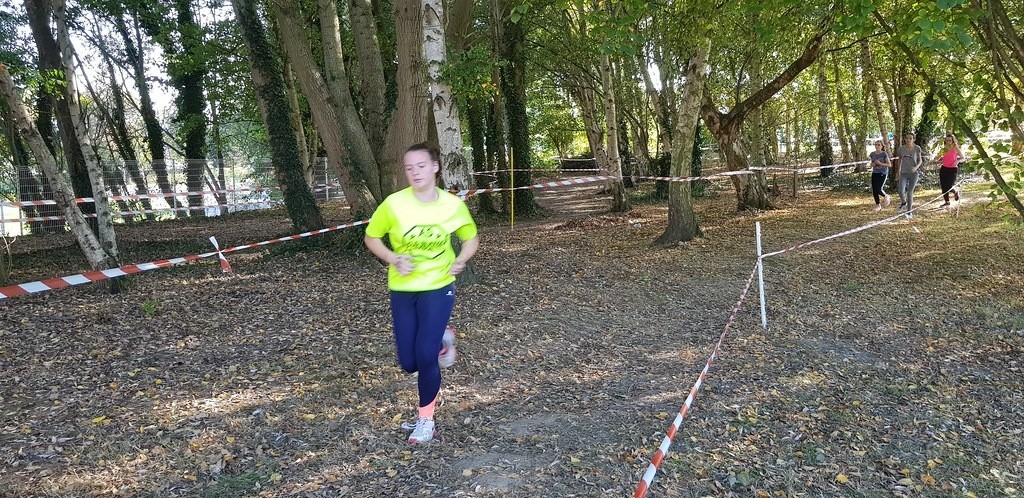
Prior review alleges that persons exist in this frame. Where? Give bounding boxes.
[934,134,965,207]
[896,132,923,218]
[364,141,480,444]
[870,140,892,212]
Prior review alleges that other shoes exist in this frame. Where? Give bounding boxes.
[896,202,908,211]
[872,204,882,212]
[884,194,890,208]
[940,201,950,208]
[906,212,913,219]
[954,190,960,201]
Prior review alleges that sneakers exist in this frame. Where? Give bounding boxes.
[438,326,457,368]
[408,417,438,444]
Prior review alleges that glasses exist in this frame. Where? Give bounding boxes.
[875,144,880,146]
